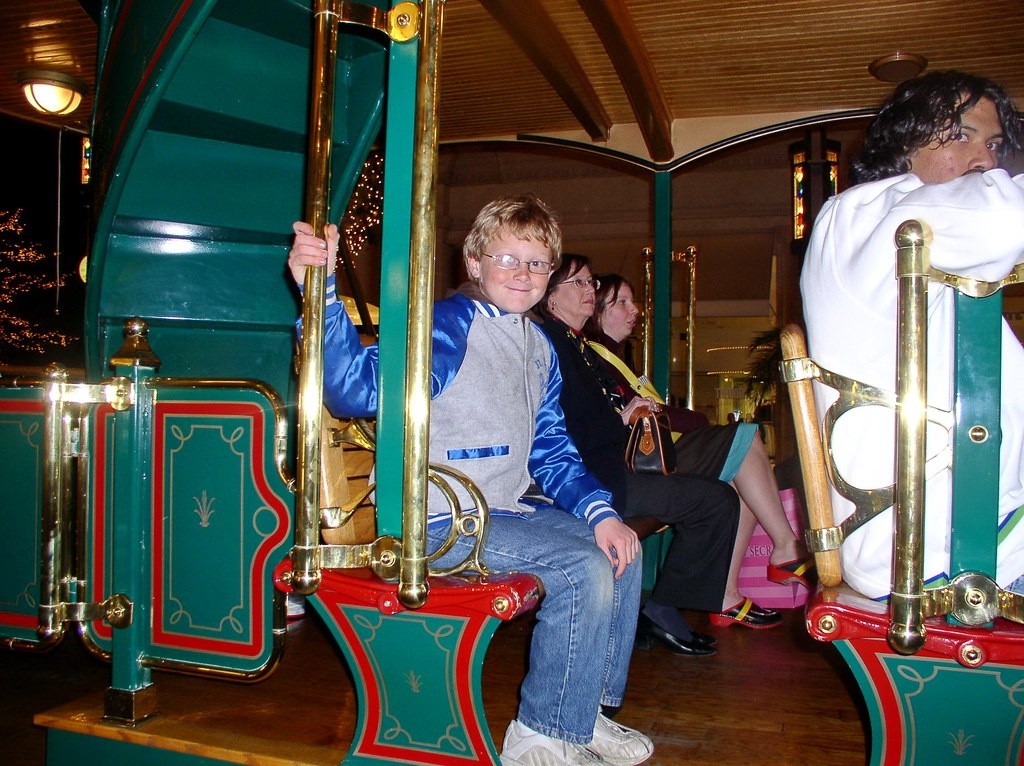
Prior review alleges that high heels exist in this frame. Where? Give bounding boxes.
[634,607,719,655]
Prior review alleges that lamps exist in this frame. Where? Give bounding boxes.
[16,68,89,118]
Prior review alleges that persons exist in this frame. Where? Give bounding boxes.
[531,253,817,658]
[802,71,1024,595]
[286,194,653,766]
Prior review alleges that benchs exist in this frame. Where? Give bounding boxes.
[775,321,1024,766]
[273,321,668,766]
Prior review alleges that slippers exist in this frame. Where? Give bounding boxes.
[766,552,819,589]
[709,595,783,630]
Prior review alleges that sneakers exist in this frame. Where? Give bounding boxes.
[499,719,613,766]
[583,705,653,766]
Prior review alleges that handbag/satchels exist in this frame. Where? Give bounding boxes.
[625,405,677,477]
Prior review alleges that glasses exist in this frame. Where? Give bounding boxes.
[552,278,601,291]
[481,254,556,274]
